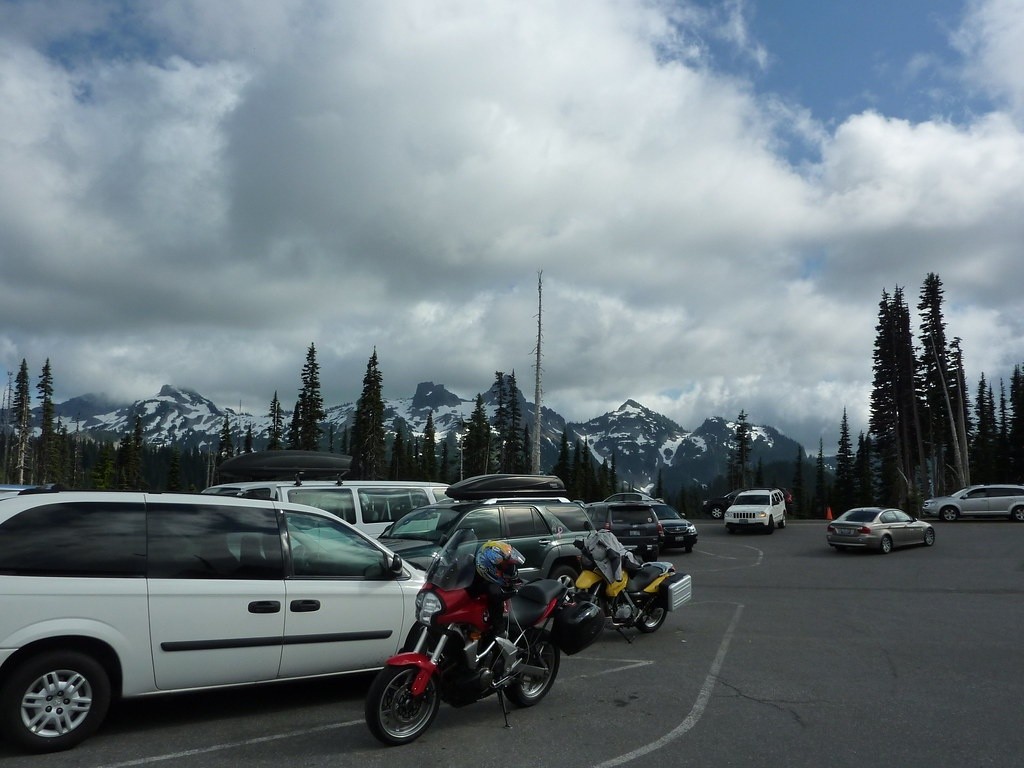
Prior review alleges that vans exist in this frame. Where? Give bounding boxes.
[0,490,426,754]
[199,449,452,562]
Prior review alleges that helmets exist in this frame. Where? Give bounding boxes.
[475,541,525,593]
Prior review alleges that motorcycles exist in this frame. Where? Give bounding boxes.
[364,528,605,747]
[567,521,693,644]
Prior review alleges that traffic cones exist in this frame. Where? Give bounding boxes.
[826,507,833,519]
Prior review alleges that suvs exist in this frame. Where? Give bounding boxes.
[724,488,787,535]
[376,474,598,617]
[621,500,698,553]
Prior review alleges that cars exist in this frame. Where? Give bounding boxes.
[702,489,792,519]
[921,484,1024,522]
[584,502,665,563]
[587,493,664,504]
[826,507,935,555]
